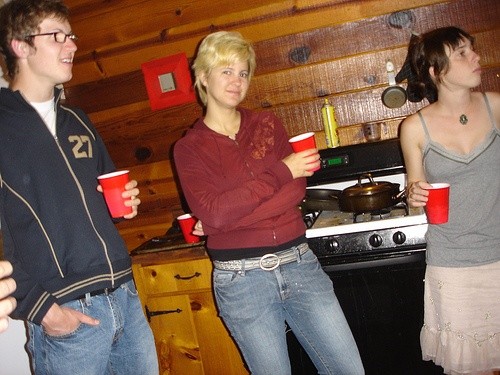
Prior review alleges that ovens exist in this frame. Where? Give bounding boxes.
[284,243,446,375]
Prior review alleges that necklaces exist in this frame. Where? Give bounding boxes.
[460,114,468,124]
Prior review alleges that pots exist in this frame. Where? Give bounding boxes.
[300,188,342,211]
[338,172,407,212]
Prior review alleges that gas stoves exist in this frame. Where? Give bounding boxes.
[302,138,433,258]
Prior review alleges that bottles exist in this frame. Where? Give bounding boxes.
[320,99,340,149]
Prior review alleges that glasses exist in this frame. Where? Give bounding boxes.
[25,31,79,44]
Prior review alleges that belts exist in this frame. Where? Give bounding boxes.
[72,285,120,300]
[212,242,309,271]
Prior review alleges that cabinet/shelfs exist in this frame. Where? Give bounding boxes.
[128,247,250,375]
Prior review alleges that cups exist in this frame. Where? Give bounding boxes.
[361,121,387,143]
[177,214,200,243]
[96,169,133,218]
[289,132,320,172]
[422,183,450,223]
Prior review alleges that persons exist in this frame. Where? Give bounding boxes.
[401,26,500,375]
[0,0,159,375]
[173,31,365,375]
